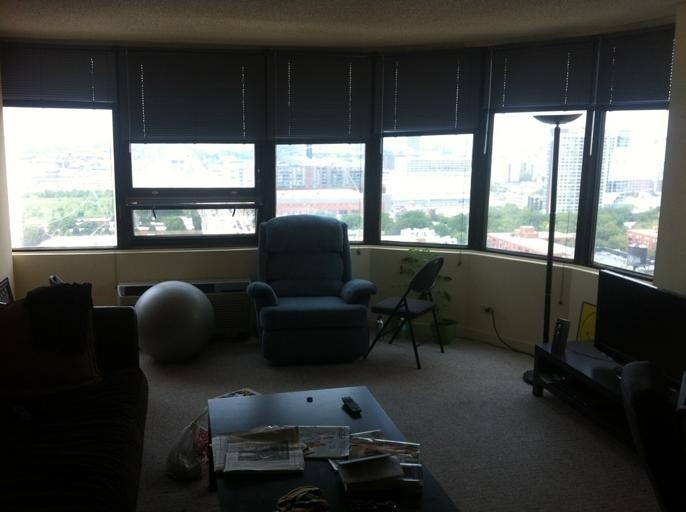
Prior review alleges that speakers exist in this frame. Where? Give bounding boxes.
[551,317,570,355]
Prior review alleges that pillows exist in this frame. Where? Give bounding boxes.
[20,281,105,392]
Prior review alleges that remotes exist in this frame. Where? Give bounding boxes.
[341,396,363,413]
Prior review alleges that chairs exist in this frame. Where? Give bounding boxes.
[244,215,445,370]
[619,359,686,512]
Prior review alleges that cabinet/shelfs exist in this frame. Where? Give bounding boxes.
[530,338,676,456]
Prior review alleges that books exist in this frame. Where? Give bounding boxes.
[336,452,405,493]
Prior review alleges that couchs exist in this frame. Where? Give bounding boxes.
[2,296,150,510]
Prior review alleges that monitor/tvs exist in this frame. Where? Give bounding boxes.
[594,268,686,390]
